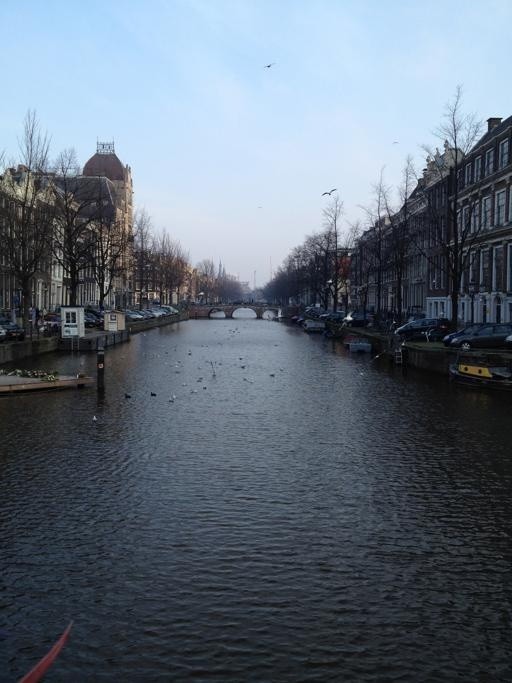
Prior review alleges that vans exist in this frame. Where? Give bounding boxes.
[394,317,512,352]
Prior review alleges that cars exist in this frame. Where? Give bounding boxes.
[0,304,178,344]
[304,303,375,326]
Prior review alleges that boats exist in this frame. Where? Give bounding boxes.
[447,352,512,388]
